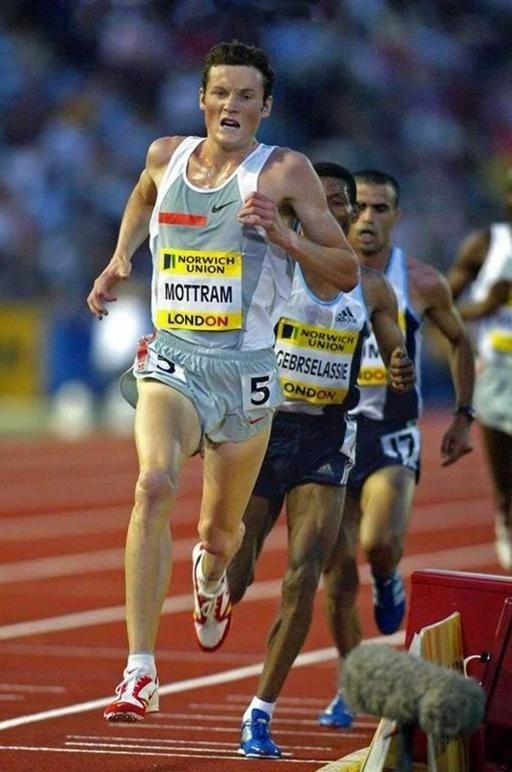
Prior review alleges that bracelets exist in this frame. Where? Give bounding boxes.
[451,403,473,424]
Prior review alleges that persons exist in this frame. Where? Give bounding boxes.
[319,171,476,726]
[86,39,361,722]
[447,179,511,579]
[228,162,419,760]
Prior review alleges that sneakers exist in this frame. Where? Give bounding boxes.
[319,692,354,727]
[190,541,233,653]
[103,665,160,724]
[237,708,281,759]
[494,523,512,571]
[370,571,406,636]
